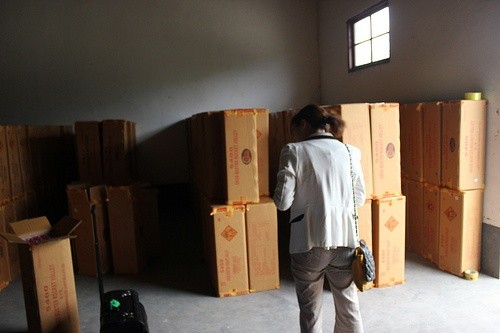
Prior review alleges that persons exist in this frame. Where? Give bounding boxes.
[274,103,367,333]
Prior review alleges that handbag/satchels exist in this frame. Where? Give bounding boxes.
[351,240,375,292]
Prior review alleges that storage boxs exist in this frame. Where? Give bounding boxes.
[0,100,488,333]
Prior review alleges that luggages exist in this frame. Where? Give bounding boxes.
[90,204,149,333]
